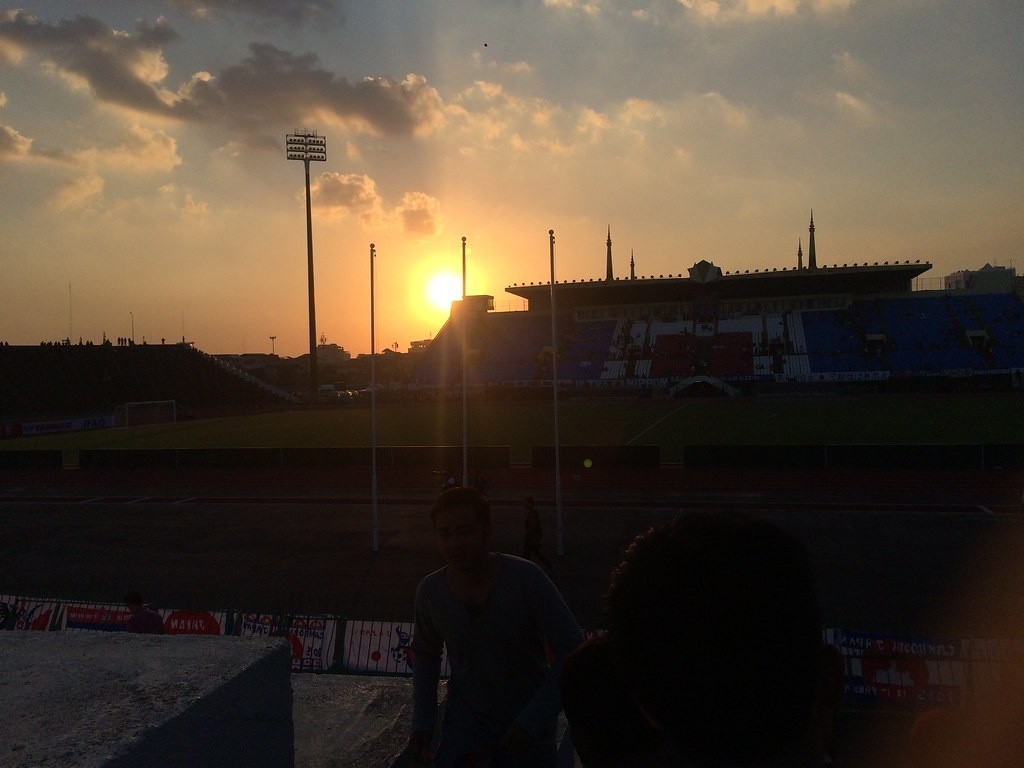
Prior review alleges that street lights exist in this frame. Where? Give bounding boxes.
[129,311,134,342]
[269,336,277,354]
[285,126,328,406]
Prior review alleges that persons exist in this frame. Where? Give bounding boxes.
[598,510,844,768]
[410,487,582,768]
[127,593,165,635]
[910,659,1003,768]
[524,497,548,564]
